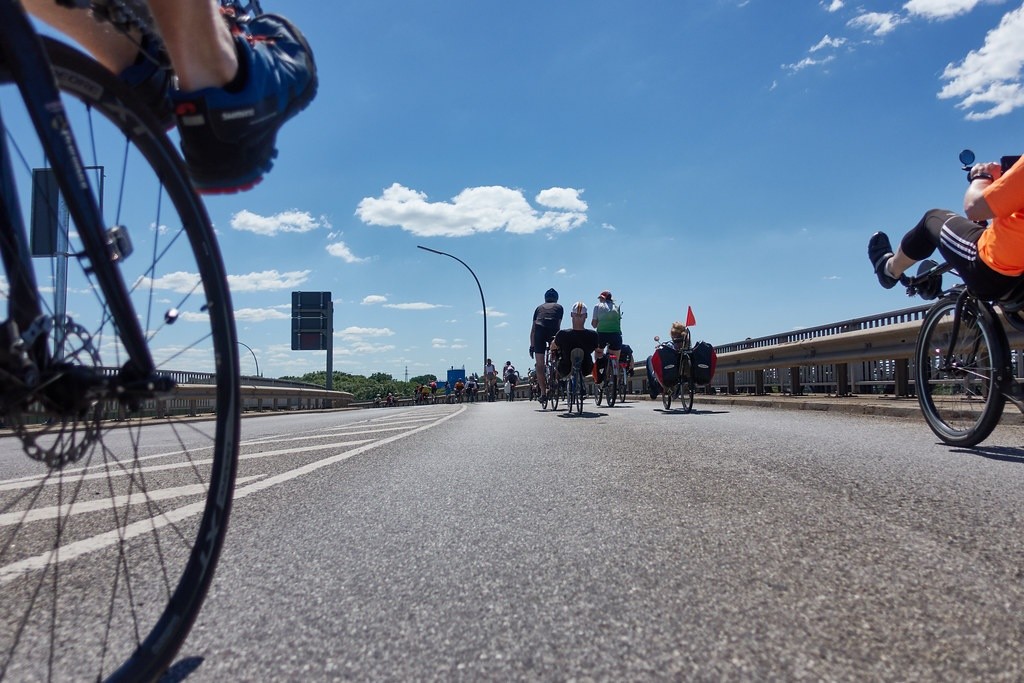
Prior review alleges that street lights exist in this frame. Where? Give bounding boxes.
[417,244,488,401]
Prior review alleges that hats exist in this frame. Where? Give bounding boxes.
[571,302,589,315]
[545,287,559,301]
[597,290,614,300]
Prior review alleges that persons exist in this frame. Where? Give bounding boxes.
[18,0,319,195]
[503,361,515,397]
[385,393,395,406]
[373,394,382,405]
[529,363,542,401]
[444,381,452,398]
[465,376,477,392]
[868,153,1024,300]
[550,302,599,400]
[602,344,635,389]
[414,383,429,403]
[529,288,563,402]
[474,378,480,391]
[484,359,495,393]
[646,322,690,400]
[591,291,622,384]
[429,380,437,403]
[454,378,465,398]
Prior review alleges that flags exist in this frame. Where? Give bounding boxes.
[686,306,696,327]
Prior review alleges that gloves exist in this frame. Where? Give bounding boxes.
[529,346,537,360]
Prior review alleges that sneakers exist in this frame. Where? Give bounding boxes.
[173,12,321,199]
[117,36,177,133]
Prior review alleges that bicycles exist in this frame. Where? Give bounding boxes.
[660,347,697,413]
[900,147,1024,448]
[482,333,629,416]
[0,2,318,683]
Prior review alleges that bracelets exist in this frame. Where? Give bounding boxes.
[970,173,995,183]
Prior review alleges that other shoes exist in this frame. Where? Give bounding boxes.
[537,394,548,403]
[868,231,902,289]
